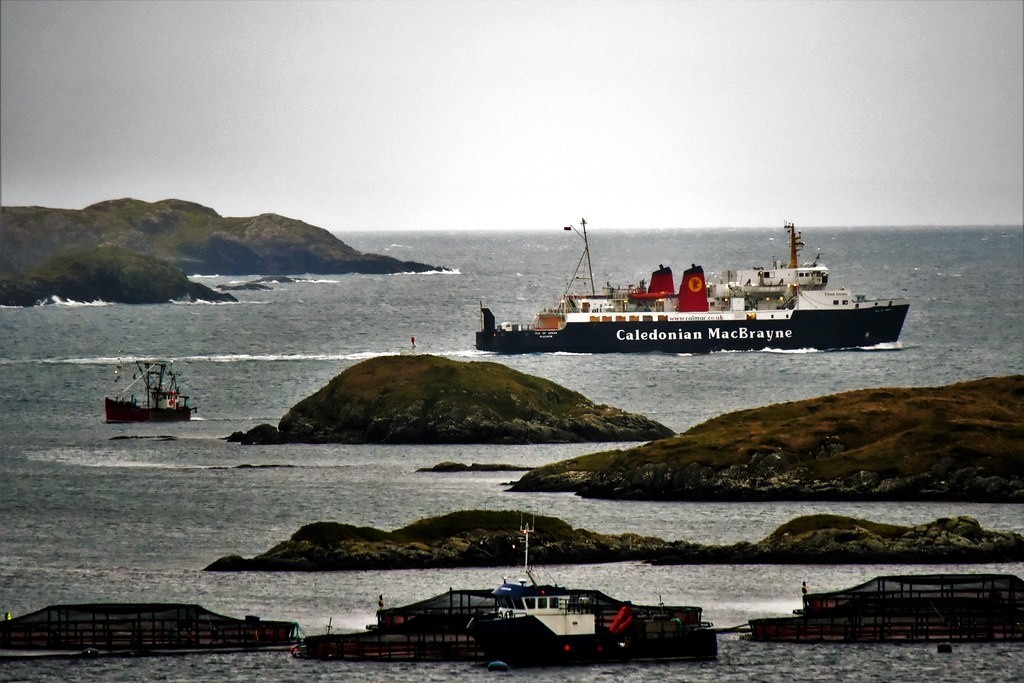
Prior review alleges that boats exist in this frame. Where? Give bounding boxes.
[103,356,198,423]
[744,572,1024,647]
[465,531,719,662]
[475,215,909,356]
